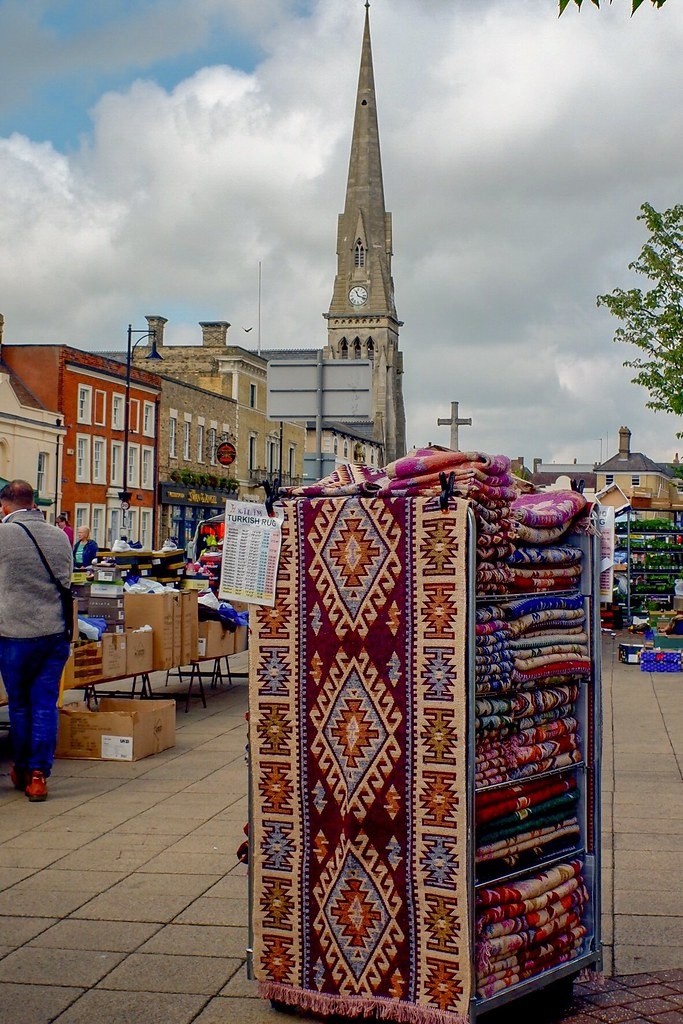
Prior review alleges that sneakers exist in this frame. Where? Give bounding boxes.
[127,540,142,548]
[162,538,177,551]
[112,540,131,552]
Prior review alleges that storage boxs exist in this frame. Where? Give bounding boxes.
[632,496,652,508]
[618,644,653,664]
[640,650,682,672]
[0,549,246,763]
[595,483,631,513]
[649,611,677,628]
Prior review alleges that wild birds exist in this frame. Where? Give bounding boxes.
[242,327,253,333]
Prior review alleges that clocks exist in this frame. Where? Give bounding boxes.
[348,286,368,306]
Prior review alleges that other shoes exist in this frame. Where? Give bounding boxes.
[24,770,48,802]
[9,764,24,789]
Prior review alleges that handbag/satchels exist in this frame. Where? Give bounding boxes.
[59,588,80,643]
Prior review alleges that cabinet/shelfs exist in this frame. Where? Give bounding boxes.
[247,507,603,1024]
[614,508,683,618]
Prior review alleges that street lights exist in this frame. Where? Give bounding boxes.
[119,324,165,538]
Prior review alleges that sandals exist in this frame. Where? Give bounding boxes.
[186,563,212,576]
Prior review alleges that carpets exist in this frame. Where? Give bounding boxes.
[237,446,604,1024]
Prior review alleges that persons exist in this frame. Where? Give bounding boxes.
[73,526,98,572]
[0,479,74,802]
[56,515,74,548]
